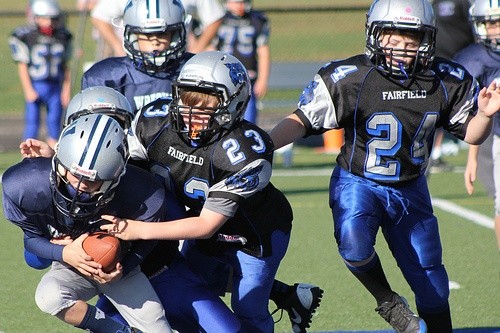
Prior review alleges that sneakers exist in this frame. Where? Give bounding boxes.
[374,291,427,333]
[271,283,325,333]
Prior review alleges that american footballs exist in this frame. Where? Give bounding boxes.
[81,232,123,274]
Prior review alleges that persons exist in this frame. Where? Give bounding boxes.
[0,0,325,333]
[429,0,500,254]
[269,0,500,333]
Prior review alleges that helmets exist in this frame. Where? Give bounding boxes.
[122,0,185,47]
[52,114,131,206]
[175,50,252,129]
[468,0,500,44]
[31,1,60,29]
[365,0,436,65]
[65,86,134,127]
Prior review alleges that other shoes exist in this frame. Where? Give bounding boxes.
[110,314,132,333]
[430,158,453,172]
